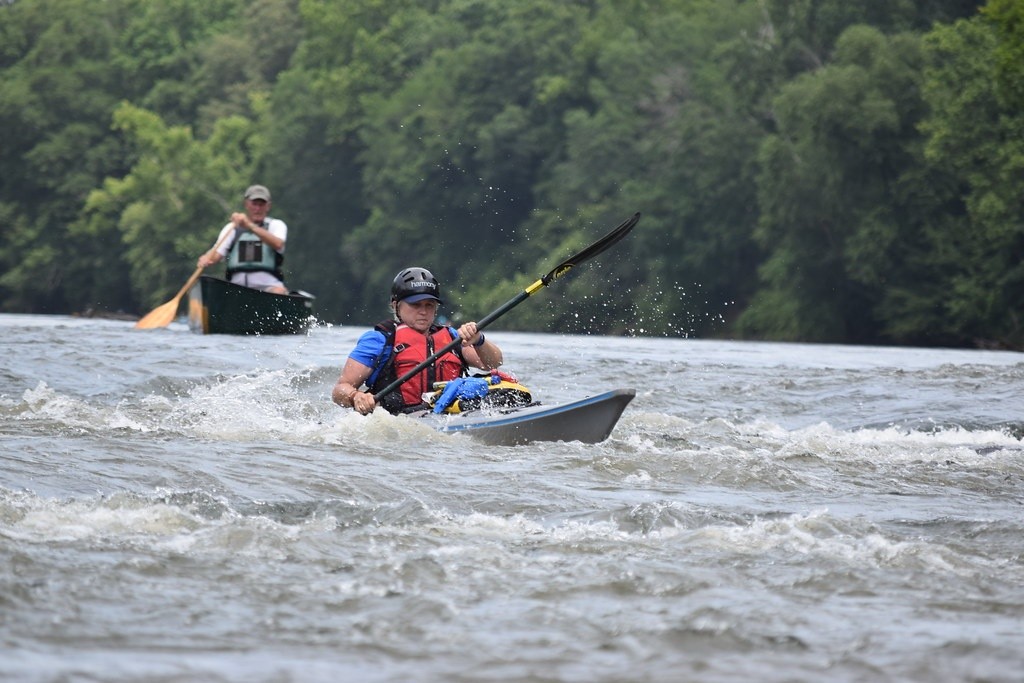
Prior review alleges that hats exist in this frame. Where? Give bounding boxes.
[245,185,271,203]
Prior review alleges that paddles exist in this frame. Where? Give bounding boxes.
[136,222,237,328]
[353,212,641,417]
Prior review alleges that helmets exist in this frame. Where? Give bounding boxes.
[391,266,443,305]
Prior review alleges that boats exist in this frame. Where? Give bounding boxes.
[186,275,316,337]
[440,387,636,448]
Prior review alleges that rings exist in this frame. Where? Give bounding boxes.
[466,324,470,328]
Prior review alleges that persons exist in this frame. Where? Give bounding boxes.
[331,266,532,421]
[197,184,288,295]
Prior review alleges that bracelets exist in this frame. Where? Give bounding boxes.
[473,333,484,347]
[349,390,360,407]
[247,223,257,234]
